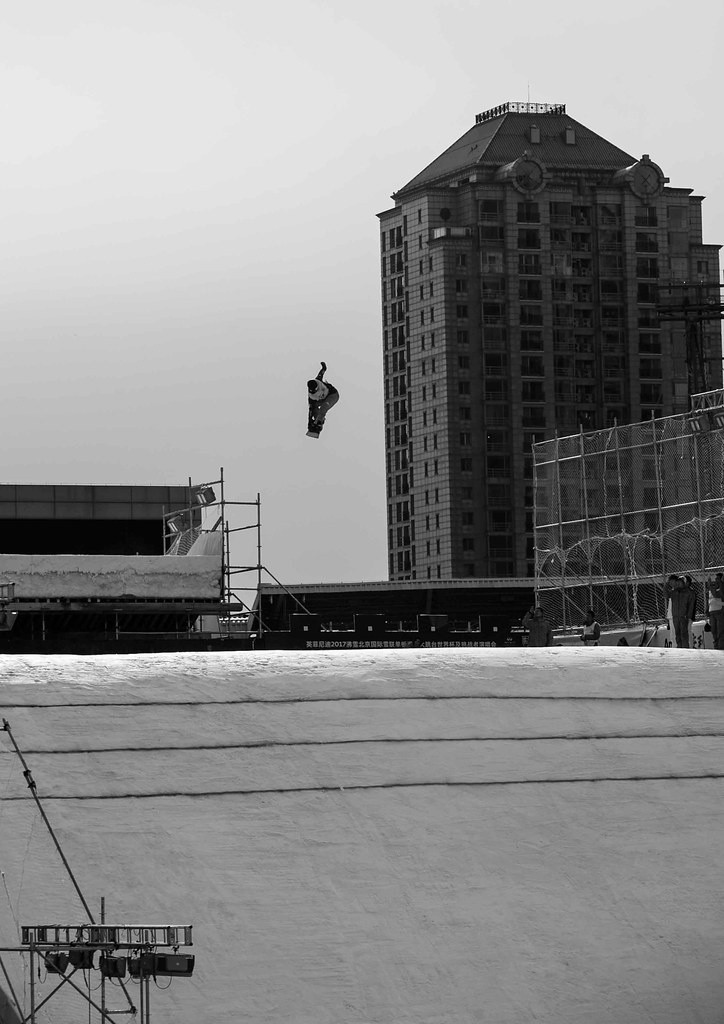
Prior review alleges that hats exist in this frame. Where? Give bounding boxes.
[685,575,692,582]
[668,574,678,580]
[677,576,686,584]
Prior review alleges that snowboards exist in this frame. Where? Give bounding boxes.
[306,412,327,439]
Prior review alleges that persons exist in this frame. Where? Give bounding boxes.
[307,361,339,431]
[705,573,724,650]
[579,611,600,646]
[522,607,553,647]
[666,575,696,648]
